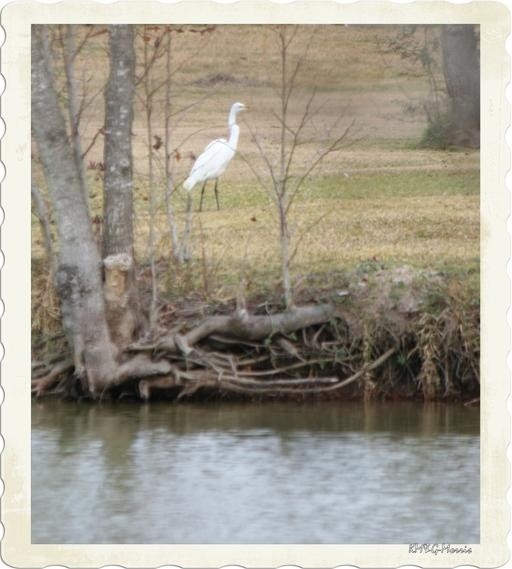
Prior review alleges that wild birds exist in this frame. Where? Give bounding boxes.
[181,101,251,212]
